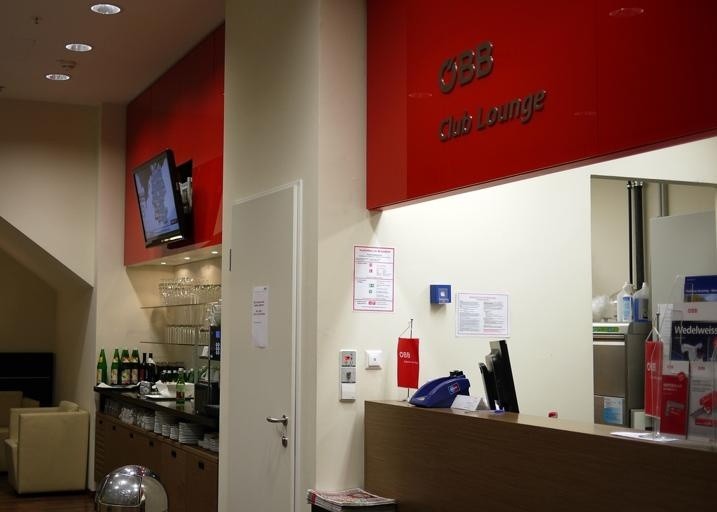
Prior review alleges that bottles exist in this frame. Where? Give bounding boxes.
[95,347,157,387]
[175,367,187,408]
[617,280,651,322]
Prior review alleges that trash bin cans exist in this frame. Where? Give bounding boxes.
[94,465,170,512]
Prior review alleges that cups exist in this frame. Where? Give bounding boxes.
[201,367,219,383]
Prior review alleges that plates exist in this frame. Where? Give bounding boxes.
[154,410,197,445]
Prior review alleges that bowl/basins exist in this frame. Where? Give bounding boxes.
[203,432,219,454]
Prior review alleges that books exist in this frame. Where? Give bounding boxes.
[306,487,396,511]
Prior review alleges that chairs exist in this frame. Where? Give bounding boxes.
[1,390,90,495]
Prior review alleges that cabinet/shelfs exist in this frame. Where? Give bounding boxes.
[94,411,219,512]
[138,302,208,347]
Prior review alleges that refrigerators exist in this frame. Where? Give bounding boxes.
[593,323,654,432]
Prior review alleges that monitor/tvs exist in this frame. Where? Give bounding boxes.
[479,339,520,413]
[132,149,188,248]
